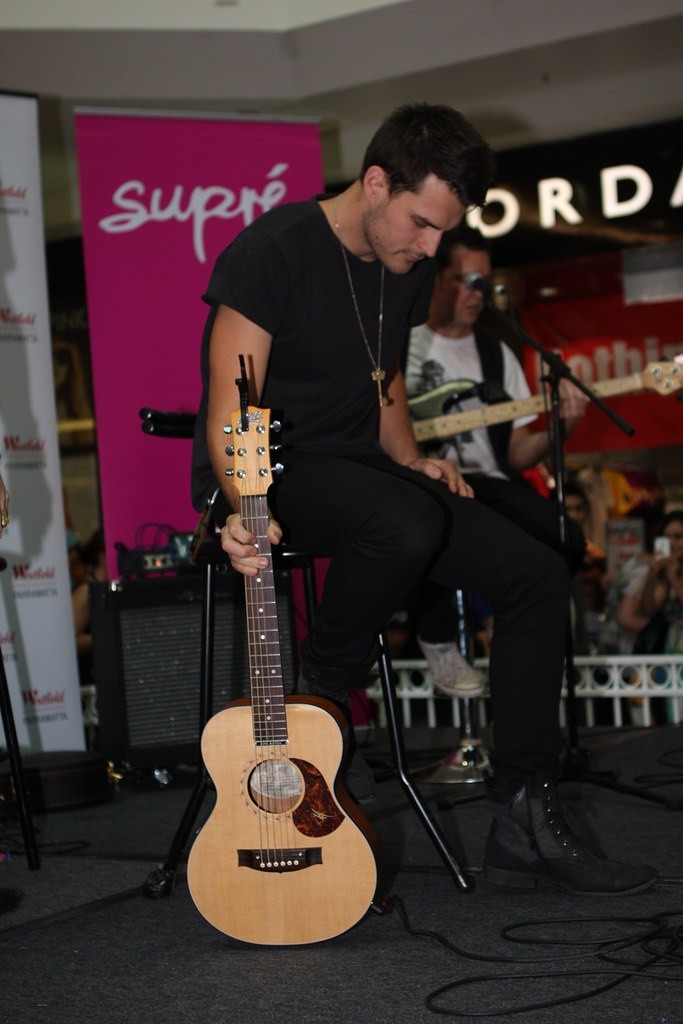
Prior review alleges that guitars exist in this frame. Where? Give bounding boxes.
[407,349,683,475]
[184,354,378,947]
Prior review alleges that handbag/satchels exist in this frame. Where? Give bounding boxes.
[632,599,667,656]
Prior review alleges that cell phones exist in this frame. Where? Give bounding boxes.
[654,535,670,558]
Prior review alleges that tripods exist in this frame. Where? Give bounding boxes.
[435,313,683,818]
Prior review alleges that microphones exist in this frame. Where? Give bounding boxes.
[466,271,504,322]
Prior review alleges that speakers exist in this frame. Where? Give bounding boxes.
[90,575,298,771]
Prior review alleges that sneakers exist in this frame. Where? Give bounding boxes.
[479,768,658,896]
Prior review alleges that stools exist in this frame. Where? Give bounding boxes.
[131,402,415,933]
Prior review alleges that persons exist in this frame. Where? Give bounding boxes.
[203,95,661,900]
[600,506,672,658]
[631,509,683,658]
[401,225,600,699]
[561,483,619,623]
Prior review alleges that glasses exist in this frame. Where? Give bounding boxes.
[566,504,583,511]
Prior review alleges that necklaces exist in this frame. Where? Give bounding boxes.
[326,193,410,409]
